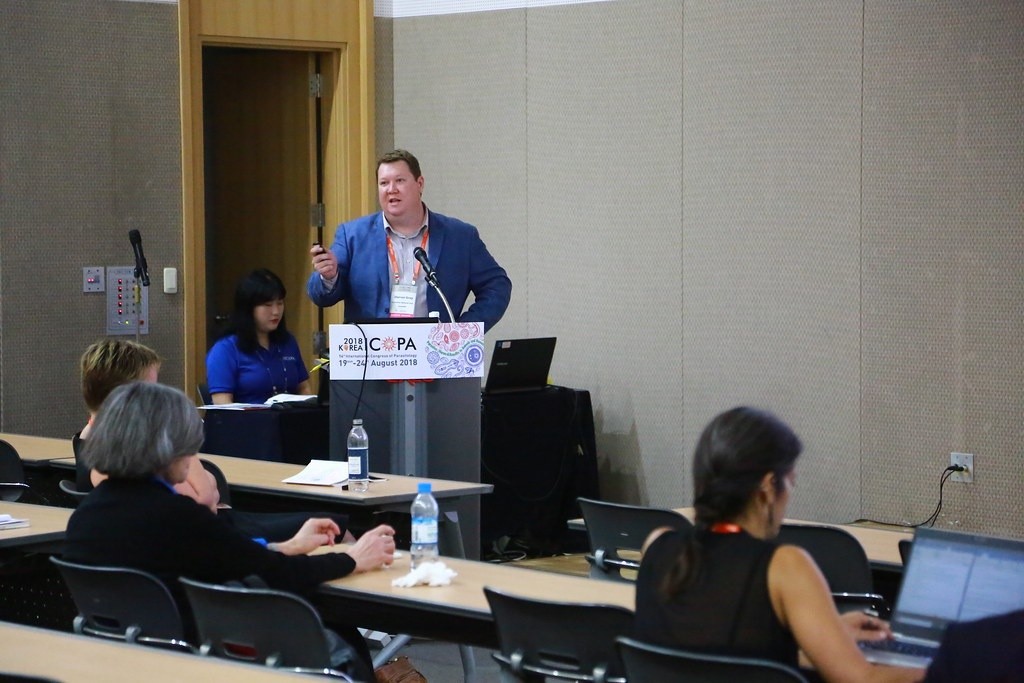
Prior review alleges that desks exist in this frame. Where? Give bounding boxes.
[1,621,345,682]
[567,505,918,600]
[22,451,495,682]
[198,385,603,556]
[1,499,87,551]
[0,428,86,488]
[307,538,812,682]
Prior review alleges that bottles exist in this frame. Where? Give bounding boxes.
[410,483,438,571]
[429,311,442,324]
[347,419,369,493]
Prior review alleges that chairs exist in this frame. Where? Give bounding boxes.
[0,437,871,682]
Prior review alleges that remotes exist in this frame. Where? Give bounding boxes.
[314,242,327,261]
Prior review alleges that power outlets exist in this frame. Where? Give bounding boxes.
[949,448,975,485]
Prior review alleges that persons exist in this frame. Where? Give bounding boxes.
[205,268,311,405]
[67,380,395,683]
[72,338,221,506]
[630,406,930,683]
[306,149,514,335]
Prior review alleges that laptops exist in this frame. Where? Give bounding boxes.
[479,337,558,396]
[863,526,1024,669]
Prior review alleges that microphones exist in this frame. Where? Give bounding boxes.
[413,247,439,286]
[129,229,151,286]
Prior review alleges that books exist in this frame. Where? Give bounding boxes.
[0,514,30,530]
[282,460,388,487]
